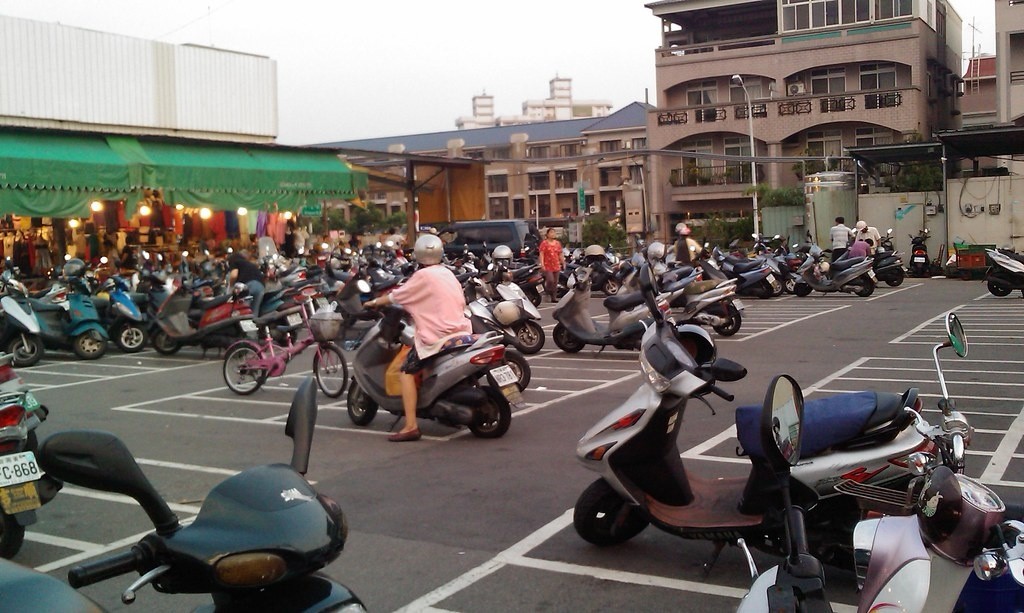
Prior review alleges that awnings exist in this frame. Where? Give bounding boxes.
[0,124,371,219]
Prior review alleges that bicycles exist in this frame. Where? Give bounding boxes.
[224,289,350,399]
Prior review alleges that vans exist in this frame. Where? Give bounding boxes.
[440,220,547,263]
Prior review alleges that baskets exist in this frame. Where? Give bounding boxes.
[309,317,343,341]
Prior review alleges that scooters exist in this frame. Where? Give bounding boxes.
[0,347,66,562]
[0,372,368,613]
[343,298,524,439]
[905,228,932,277]
[980,248,1024,299]
[572,259,937,577]
[0,229,908,368]
[723,309,1024,613]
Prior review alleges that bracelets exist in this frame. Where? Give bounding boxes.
[373,298,377,307]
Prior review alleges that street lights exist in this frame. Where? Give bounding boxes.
[732,75,760,243]
[580,157,607,223]
[526,185,540,231]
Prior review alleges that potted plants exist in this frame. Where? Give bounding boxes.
[793,149,816,180]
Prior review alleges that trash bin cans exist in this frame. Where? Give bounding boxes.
[968,244,996,281]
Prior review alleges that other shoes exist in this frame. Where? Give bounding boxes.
[388,427,423,442]
[550,299,559,303]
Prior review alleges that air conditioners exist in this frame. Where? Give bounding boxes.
[589,205,601,213]
[788,83,806,96]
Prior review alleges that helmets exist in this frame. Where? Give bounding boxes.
[64,258,85,278]
[493,301,522,328]
[401,262,415,276]
[647,242,665,260]
[820,262,832,275]
[384,235,406,252]
[585,245,608,264]
[414,233,444,266]
[856,221,867,231]
[675,222,687,232]
[492,245,514,267]
[679,228,691,236]
[877,247,885,253]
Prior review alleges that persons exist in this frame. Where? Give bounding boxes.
[363,235,472,441]
[539,228,566,303]
[856,221,881,248]
[119,236,135,268]
[673,222,703,281]
[73,227,87,259]
[349,232,361,247]
[249,234,257,245]
[103,240,120,264]
[828,150,838,170]
[829,216,855,262]
[385,228,407,245]
[312,232,329,251]
[229,252,264,343]
